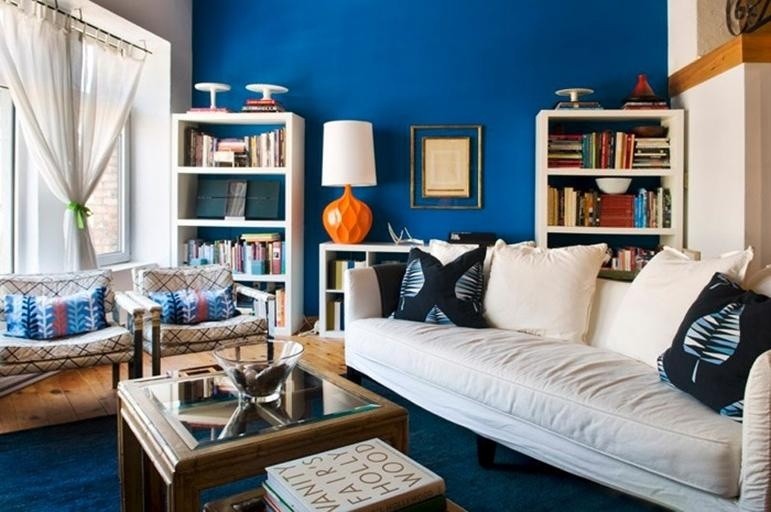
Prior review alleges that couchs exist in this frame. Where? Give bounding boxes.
[340,241,770,512]
[0,269,143,433]
[126,264,277,379]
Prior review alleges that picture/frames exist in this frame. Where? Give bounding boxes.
[409,121,486,214]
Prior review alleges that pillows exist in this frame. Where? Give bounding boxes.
[401,247,488,329]
[4,285,104,334]
[656,270,754,416]
[594,243,754,369]
[152,283,234,322]
[477,239,605,338]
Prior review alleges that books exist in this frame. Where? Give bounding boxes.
[328,260,365,290]
[622,94,669,110]
[335,302,340,331]
[252,287,286,330]
[635,137,670,168]
[584,132,634,170]
[634,187,671,228]
[185,107,229,114]
[327,302,334,331]
[261,437,449,512]
[242,105,282,111]
[183,232,285,276]
[548,134,584,168]
[611,245,654,272]
[183,125,286,167]
[548,186,601,227]
[246,99,276,104]
[554,100,603,110]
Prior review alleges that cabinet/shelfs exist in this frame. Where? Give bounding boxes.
[535,109,685,250]
[316,242,429,338]
[171,112,306,335]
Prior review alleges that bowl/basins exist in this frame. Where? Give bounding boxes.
[212,337,306,406]
[596,178,632,195]
[634,126,666,138]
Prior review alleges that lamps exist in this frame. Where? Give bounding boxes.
[317,120,377,242]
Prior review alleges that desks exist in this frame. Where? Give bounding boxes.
[202,477,472,512]
